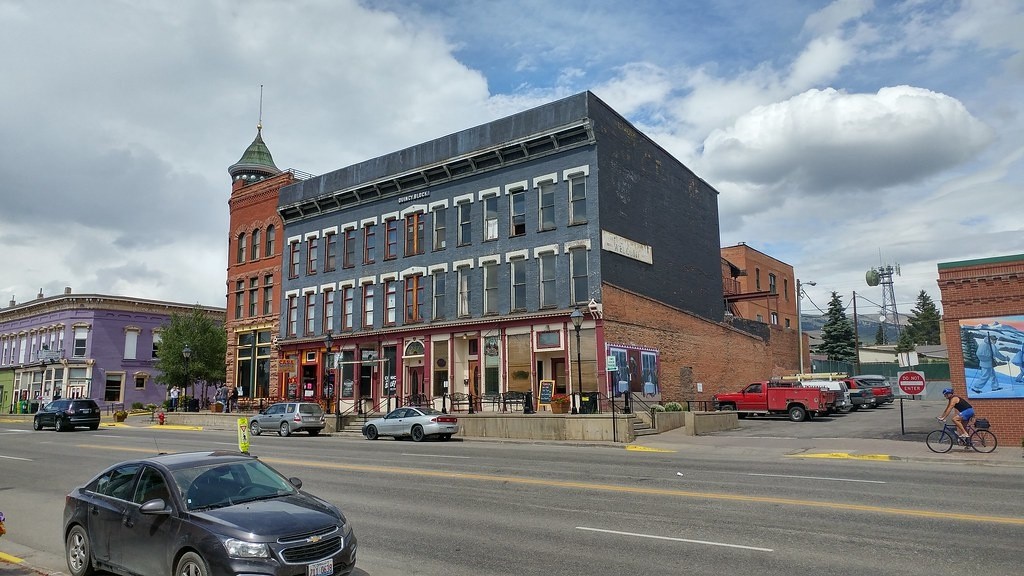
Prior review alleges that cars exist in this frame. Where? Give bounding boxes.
[772,371,895,417]
[362,406,460,443]
[63,448,359,576]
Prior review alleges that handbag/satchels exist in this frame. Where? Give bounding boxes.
[974,418,990,428]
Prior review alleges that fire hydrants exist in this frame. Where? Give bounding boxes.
[158,411,165,426]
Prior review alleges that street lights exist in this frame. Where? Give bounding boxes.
[797,279,818,375]
[570,306,587,415]
[323,332,335,414]
[182,345,192,414]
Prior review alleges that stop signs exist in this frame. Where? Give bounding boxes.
[899,371,925,395]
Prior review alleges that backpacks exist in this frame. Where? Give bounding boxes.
[976,343,993,361]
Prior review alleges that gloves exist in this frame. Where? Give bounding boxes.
[1005,357,1010,362]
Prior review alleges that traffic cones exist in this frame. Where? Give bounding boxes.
[39,359,48,408]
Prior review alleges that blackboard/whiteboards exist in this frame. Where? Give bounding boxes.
[538,380,556,405]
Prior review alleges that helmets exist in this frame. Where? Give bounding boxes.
[943,387,954,395]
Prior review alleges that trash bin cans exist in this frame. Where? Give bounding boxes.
[188,398,200,412]
[28,403,39,414]
[580,392,598,414]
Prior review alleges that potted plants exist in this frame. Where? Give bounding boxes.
[113,411,128,422]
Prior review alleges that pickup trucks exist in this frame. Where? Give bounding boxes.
[712,380,828,421]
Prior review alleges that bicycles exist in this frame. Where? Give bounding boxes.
[926,416,998,454]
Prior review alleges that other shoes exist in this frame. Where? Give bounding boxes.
[992,387,1002,391]
[964,445,972,449]
[959,434,970,437]
[971,388,983,394]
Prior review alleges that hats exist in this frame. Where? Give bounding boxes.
[990,335,998,340]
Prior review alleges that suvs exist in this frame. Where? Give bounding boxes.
[33,397,102,432]
[248,402,326,438]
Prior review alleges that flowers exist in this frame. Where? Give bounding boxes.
[551,393,570,403]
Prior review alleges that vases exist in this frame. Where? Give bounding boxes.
[551,402,570,414]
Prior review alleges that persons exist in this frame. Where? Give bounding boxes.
[170,386,181,407]
[937,389,974,445]
[214,383,240,412]
[972,335,1024,394]
